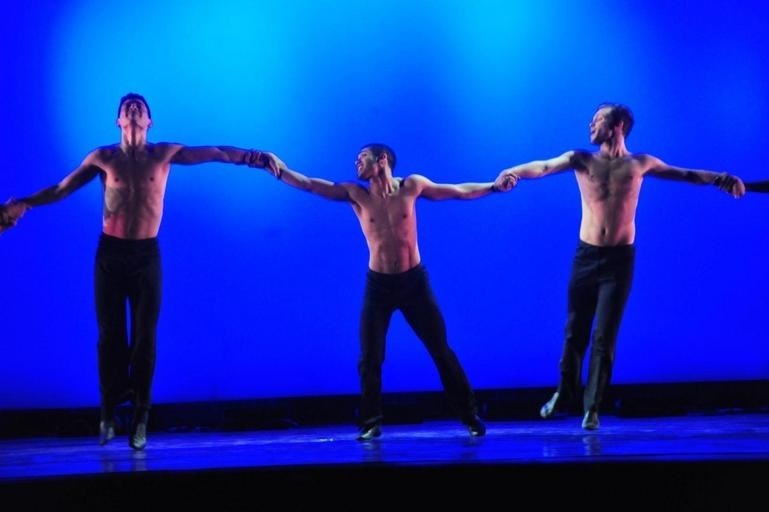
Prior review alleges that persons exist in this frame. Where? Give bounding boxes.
[245,143,520,442]
[2,93,286,450]
[495,104,746,430]
[0,202,17,234]
[712,171,769,193]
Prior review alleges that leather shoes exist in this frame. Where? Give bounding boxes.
[539,389,576,418]
[582,409,600,431]
[356,424,382,441]
[129,422,148,452]
[467,414,488,438]
[97,418,118,446]
